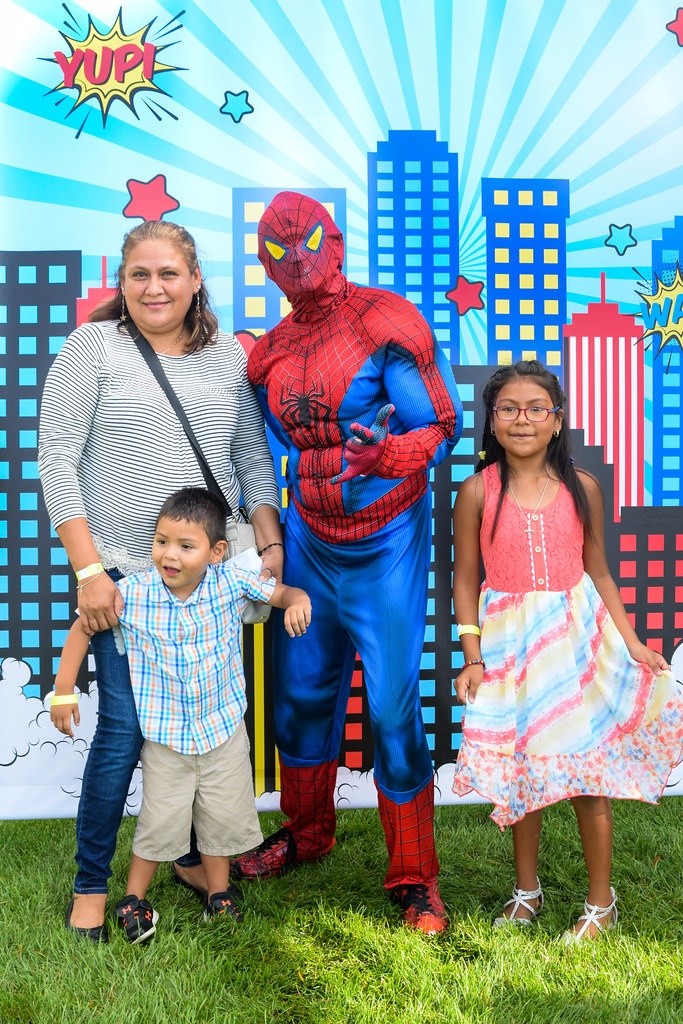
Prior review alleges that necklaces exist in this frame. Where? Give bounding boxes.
[158,331,186,356]
[507,467,552,539]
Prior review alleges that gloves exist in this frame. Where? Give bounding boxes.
[330,403,395,485]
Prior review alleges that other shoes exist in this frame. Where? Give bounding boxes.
[171,861,244,907]
[65,896,108,946]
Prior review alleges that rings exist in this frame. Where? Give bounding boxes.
[263,567,273,577]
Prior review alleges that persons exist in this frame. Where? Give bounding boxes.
[450,361,683,947]
[50,488,312,944]
[231,191,465,936]
[39,220,284,944]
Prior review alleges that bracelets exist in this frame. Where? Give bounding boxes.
[457,624,480,639]
[460,660,485,672]
[50,694,78,706]
[257,543,284,557]
[75,563,105,590]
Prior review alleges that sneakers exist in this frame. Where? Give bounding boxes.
[207,892,244,923]
[389,879,449,934]
[113,894,160,945]
[229,823,333,881]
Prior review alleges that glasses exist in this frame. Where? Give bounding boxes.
[492,406,561,422]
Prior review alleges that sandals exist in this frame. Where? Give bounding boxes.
[493,876,544,930]
[561,887,618,944]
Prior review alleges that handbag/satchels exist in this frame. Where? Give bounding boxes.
[223,520,272,621]
